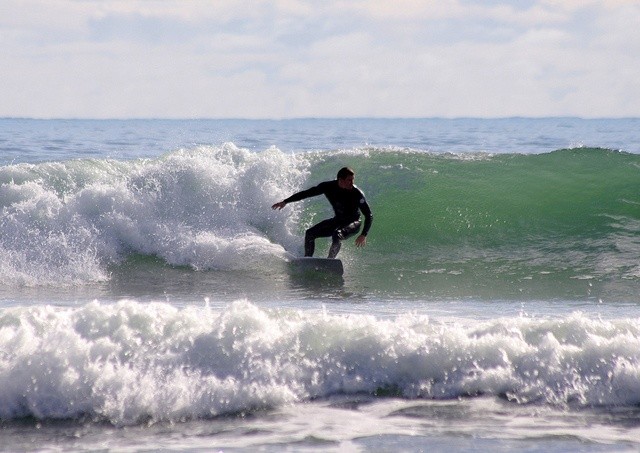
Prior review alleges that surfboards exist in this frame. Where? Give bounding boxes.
[292,257,344,275]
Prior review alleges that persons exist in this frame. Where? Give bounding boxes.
[271,167,373,258]
